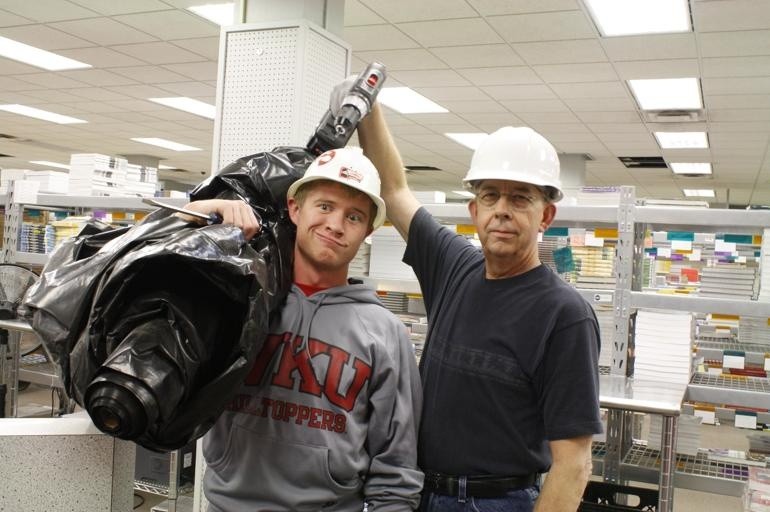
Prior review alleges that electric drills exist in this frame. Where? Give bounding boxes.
[306,61,387,157]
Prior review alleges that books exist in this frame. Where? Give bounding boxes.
[743,466,769,510]
[629,231,756,385]
[537,227,619,291]
[371,226,456,364]
[0,150,189,260]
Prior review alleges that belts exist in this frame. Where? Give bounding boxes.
[425,471,536,499]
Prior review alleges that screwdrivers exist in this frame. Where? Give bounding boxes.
[142,197,224,225]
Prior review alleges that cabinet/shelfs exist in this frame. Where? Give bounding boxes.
[0,179,195,512]
[346,185,770,512]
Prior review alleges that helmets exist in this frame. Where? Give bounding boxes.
[287,146,387,229]
[462,127,563,204]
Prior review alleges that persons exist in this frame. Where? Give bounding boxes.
[157,141,428,511]
[328,55,604,511]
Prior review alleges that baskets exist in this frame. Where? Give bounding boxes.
[579,480,659,512]
